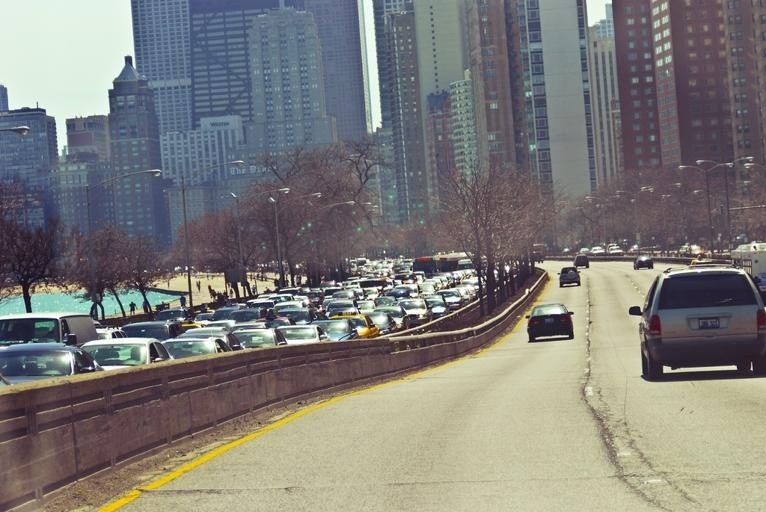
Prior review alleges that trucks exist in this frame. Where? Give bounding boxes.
[529,244,545,263]
[730,241,766,281]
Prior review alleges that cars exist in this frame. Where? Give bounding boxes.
[524,303,576,343]
[561,243,641,256]
[634,255,654,270]
[754,272,766,307]
[0,251,518,393]
[671,245,705,257]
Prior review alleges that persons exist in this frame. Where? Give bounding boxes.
[177,271,291,311]
[160,301,166,310]
[128,301,136,316]
[140,299,148,313]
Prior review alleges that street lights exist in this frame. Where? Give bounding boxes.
[696,157,756,248]
[745,162,766,176]
[575,181,703,243]
[231,187,290,298]
[679,163,733,252]
[311,199,379,288]
[271,192,321,290]
[0,125,28,136]
[181,159,245,315]
[83,167,164,319]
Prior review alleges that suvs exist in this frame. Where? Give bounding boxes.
[558,267,581,286]
[574,254,589,269]
[627,266,766,381]
